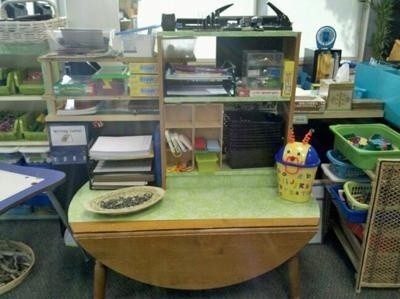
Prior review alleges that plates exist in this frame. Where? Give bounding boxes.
[83,185,165,215]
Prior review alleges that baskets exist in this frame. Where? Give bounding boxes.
[327,149,367,179]
[343,181,400,211]
[0,0,68,44]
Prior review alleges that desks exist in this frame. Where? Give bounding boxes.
[66,174,321,298]
[0,160,92,264]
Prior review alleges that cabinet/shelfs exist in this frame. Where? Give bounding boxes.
[0,26,166,219]
[155,30,303,189]
[321,141,400,294]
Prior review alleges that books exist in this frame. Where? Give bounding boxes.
[56,102,101,115]
[87,134,154,189]
